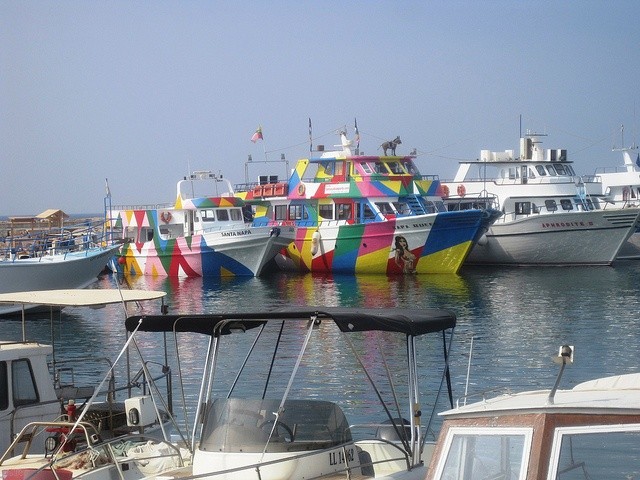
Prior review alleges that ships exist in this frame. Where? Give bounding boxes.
[440,113,639,265]
[233,115,503,273]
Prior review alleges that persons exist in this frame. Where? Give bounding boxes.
[389,235,417,273]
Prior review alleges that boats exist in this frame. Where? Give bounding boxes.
[424,343,639,480]
[0,177,123,314]
[105,158,295,276]
[70,304,455,480]
[0,287,173,459]
[591,123,638,259]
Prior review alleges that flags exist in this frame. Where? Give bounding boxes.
[251,125,263,143]
